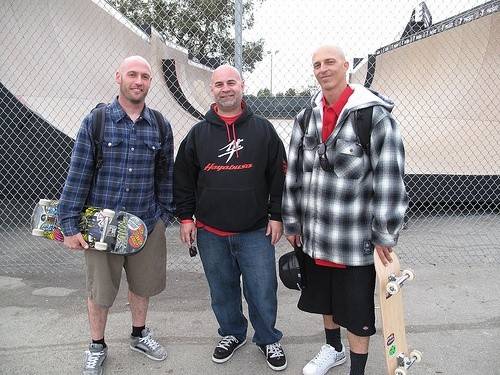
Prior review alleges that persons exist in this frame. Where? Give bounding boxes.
[282,44,408,375]
[58,56,174,375]
[173,66,288,370]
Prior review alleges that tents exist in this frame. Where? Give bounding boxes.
[0,0,500,220]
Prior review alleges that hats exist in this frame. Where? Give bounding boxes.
[279,238,306,291]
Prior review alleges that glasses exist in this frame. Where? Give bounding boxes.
[189,233,198,257]
[317,141,332,172]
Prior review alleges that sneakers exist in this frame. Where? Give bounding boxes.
[258,341,288,371]
[81,343,109,375]
[211,335,248,363]
[129,327,168,361]
[302,341,347,375]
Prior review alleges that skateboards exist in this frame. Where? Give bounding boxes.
[30,199,148,256]
[373,246,423,375]
